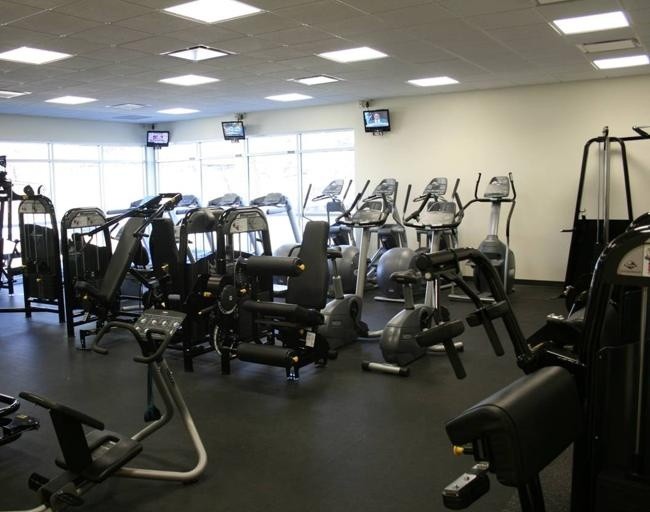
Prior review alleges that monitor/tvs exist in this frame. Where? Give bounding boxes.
[362,110,391,132]
[221,122,245,140]
[147,130,167,148]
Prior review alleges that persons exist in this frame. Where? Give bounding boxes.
[368,113,388,124]
